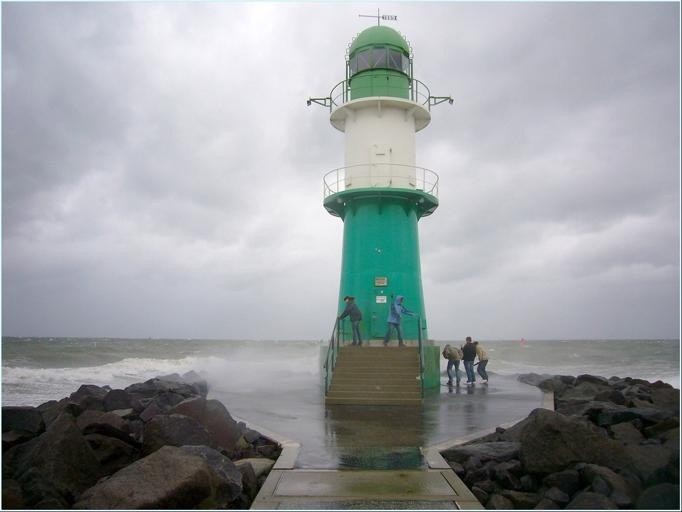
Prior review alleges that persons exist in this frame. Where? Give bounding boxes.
[338,295,363,345]
[460,336,477,385]
[471,340,489,385]
[442,344,466,387]
[382,290,418,346]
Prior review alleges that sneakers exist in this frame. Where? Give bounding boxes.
[447,379,488,385]
[383,341,407,347]
[352,341,363,346]
